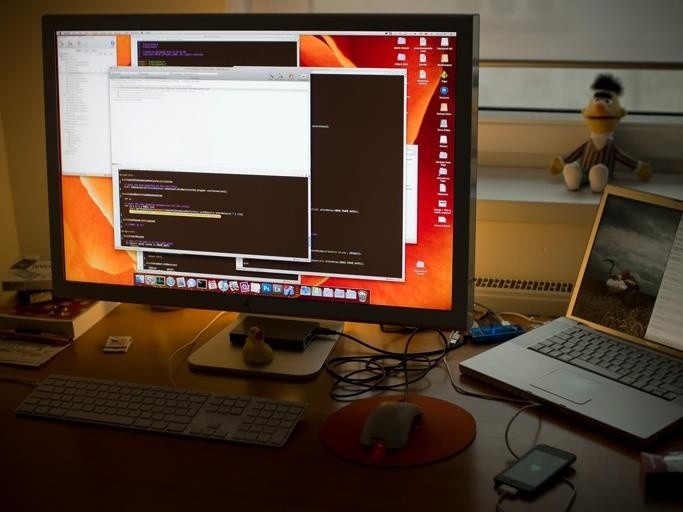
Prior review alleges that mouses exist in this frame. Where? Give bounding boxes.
[362,401,425,453]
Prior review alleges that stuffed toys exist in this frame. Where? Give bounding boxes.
[240,325,274,365]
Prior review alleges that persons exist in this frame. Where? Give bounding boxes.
[548,72,658,193]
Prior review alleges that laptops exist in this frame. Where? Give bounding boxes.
[460,186,683,440]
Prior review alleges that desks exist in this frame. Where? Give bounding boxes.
[0,304,683,512]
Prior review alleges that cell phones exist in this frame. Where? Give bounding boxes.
[494,444,577,493]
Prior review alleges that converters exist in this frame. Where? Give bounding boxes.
[471,325,524,344]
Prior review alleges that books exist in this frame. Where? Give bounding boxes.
[0,290,126,343]
[0,253,51,289]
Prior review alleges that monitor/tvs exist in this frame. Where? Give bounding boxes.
[41,14,479,382]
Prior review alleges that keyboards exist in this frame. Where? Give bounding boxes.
[16,374,309,448]
